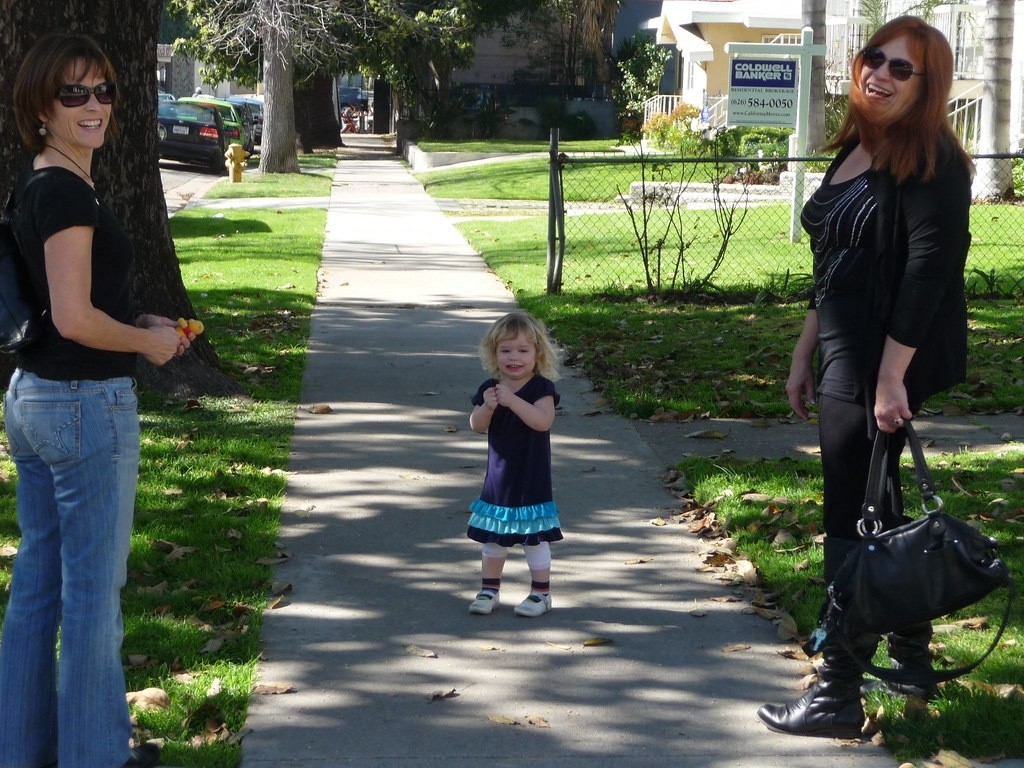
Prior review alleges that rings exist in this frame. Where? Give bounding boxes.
[894,418,902,425]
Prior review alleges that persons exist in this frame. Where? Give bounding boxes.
[755,12,977,743]
[0,30,194,768]
[340,102,373,134]
[467,310,564,616]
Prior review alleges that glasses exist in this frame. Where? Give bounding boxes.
[862,47,926,81]
[55,81,116,107]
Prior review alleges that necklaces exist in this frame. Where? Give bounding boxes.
[42,144,96,189]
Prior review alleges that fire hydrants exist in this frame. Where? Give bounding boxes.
[223,144,250,183]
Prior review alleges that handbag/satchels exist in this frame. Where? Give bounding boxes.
[802,419,1015,685]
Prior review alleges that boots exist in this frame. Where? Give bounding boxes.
[773,535,880,739]
[880,516,943,699]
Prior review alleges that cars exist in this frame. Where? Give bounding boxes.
[158,93,175,102]
[228,97,262,144]
[193,96,260,155]
[156,101,226,175]
[176,97,245,153]
[337,86,369,117]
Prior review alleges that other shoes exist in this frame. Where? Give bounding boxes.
[514,592,551,616]
[469,590,499,614]
[121,744,160,768]
